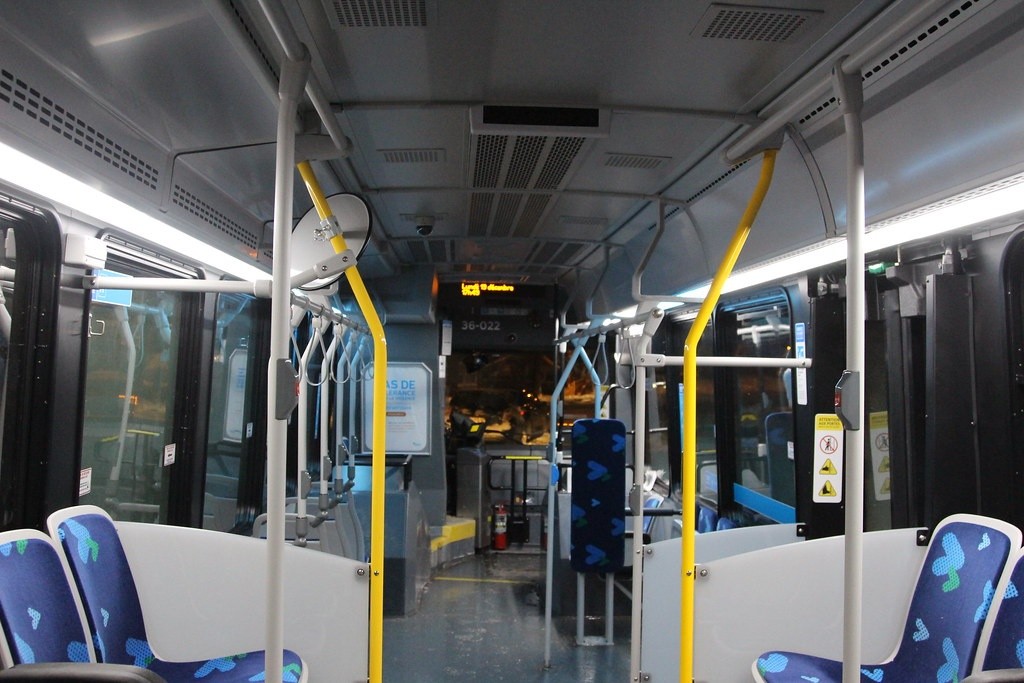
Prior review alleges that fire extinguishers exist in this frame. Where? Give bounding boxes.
[494,504,508,549]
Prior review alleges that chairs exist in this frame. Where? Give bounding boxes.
[0,527,168,683]
[748,511,1024,683]
[309,478,369,563]
[624,488,738,547]
[46,503,309,683]
